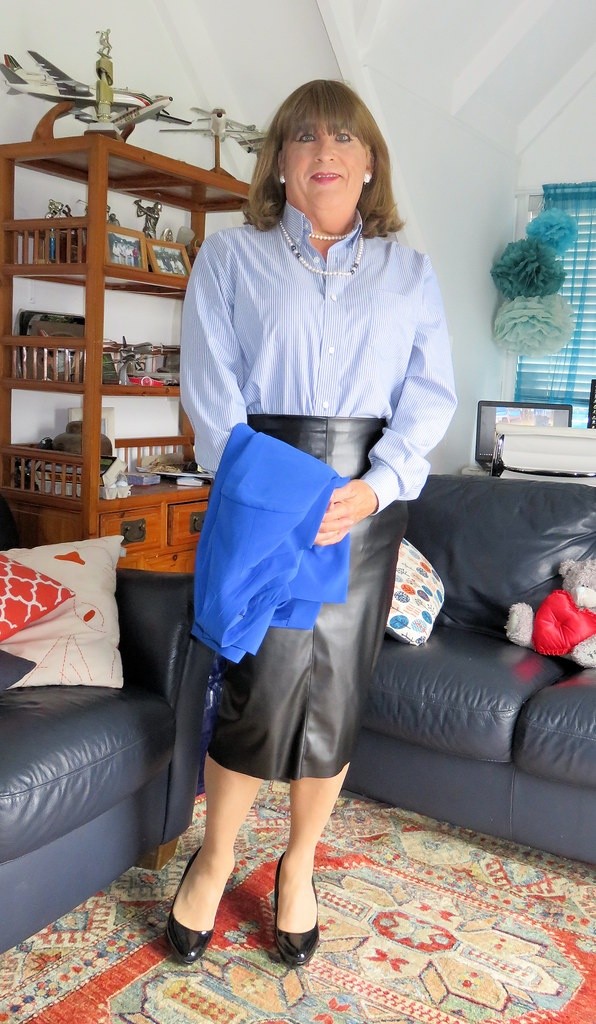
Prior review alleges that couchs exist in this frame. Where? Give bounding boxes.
[343,475,596,865]
[0,567,211,954]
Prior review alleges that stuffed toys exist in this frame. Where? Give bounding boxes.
[505,559,596,669]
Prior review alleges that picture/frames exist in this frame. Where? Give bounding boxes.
[146,238,191,276]
[105,222,149,271]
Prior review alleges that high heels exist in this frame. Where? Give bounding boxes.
[274,851,319,965]
[167,845,216,965]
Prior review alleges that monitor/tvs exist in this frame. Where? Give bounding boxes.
[475,401,572,473]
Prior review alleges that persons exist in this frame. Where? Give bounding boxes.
[166,81,459,966]
[113,240,138,267]
[133,200,162,240]
[156,252,184,275]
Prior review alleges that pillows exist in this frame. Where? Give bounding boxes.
[384,536,445,646]
[0,534,125,691]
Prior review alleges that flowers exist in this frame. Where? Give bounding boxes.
[491,207,579,355]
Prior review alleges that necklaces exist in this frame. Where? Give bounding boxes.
[280,220,364,276]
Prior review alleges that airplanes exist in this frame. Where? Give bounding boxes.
[103,335,164,356]
[0,50,191,129]
[159,106,258,138]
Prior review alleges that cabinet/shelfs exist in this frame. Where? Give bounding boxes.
[0,134,251,574]
